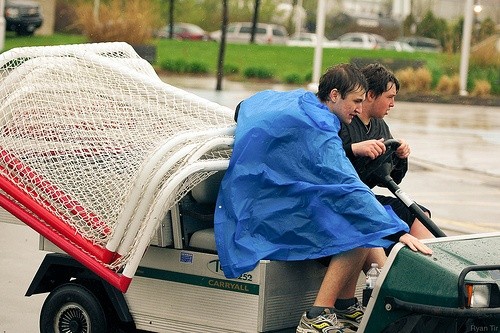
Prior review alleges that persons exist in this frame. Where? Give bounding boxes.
[214,63,433,333]
[337,64,434,281]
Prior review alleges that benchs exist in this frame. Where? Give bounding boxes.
[171,169,226,254]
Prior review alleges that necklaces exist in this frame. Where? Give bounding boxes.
[357,114,371,134]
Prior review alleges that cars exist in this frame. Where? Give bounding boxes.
[157,21,210,41]
[286,31,330,50]
[327,31,443,56]
[3,1,42,37]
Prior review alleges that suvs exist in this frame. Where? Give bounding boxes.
[207,21,290,47]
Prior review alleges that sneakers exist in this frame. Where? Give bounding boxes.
[296,310,357,333]
[334,303,365,328]
